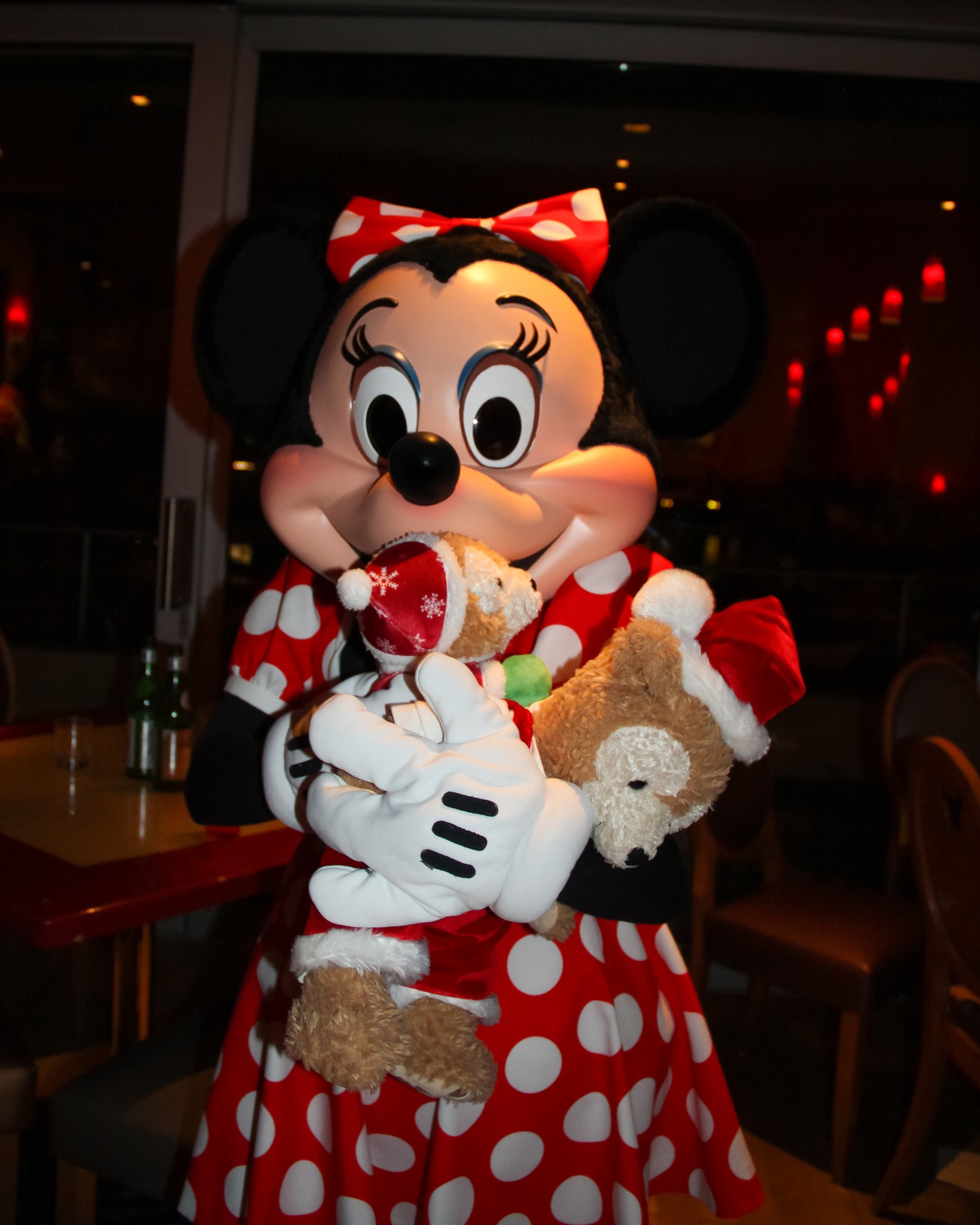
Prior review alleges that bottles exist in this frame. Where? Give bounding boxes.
[158,645,193,792]
[127,637,165,782]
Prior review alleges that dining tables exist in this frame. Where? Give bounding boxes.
[48,1004,230,1225]
[0,706,307,1058]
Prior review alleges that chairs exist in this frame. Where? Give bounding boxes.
[692,751,931,1203]
[886,655,980,858]
[867,734,980,1215]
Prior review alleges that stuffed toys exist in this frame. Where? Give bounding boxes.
[276,525,540,785]
[183,176,779,1224]
[275,564,810,1106]
